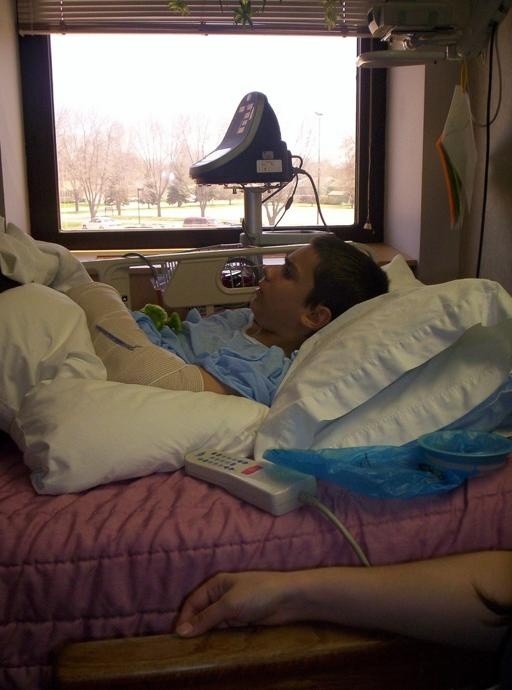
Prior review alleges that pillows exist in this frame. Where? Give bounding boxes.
[307,322,512,449]
[252,252,511,463]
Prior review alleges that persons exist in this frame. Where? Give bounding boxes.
[62,232,391,409]
[171,546,512,651]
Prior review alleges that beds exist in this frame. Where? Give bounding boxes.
[0,240,512,684]
